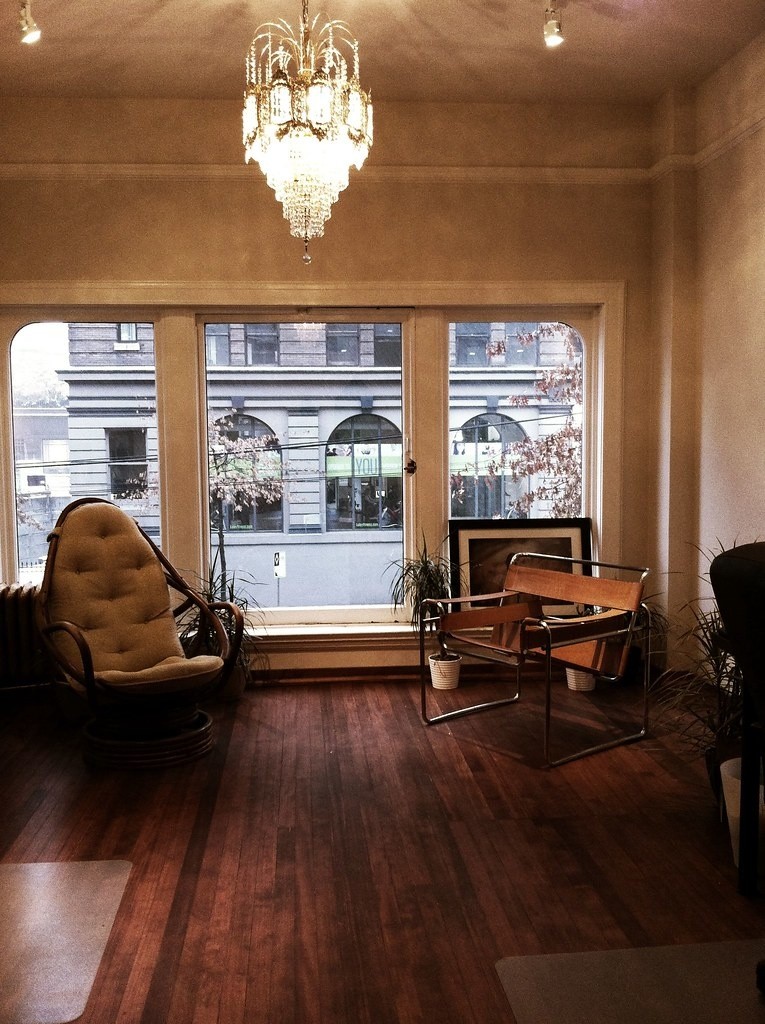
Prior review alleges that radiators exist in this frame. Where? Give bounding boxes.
[0,580,52,688]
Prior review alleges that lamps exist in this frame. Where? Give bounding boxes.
[241,0,374,265]
[542,8,565,48]
[16,0,41,46]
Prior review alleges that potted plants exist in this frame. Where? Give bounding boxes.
[625,573,672,686]
[172,540,274,705]
[641,533,762,824]
[381,526,473,690]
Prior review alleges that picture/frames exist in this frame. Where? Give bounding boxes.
[447,516,593,619]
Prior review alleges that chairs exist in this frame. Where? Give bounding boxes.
[419,552,651,767]
[711,542,765,900]
[30,497,245,771]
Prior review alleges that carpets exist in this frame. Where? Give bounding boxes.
[495,939,765,1024]
[2,860,132,1024]
